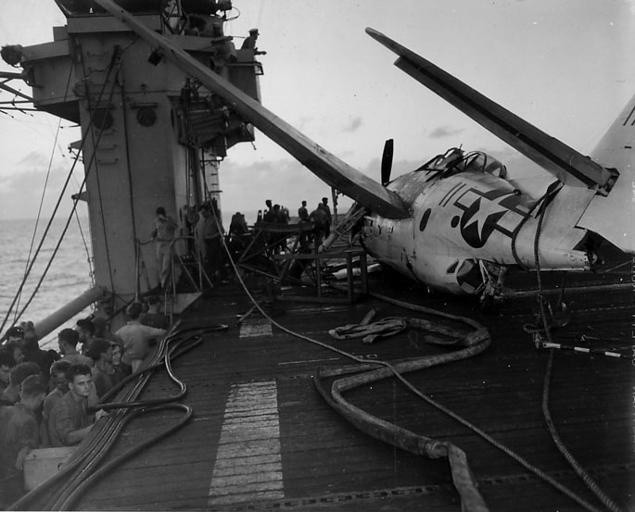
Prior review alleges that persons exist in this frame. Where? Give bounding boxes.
[151,197,333,294]
[241,28,267,56]
[0,303,168,512]
[141,296,170,329]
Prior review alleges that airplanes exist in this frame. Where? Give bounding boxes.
[96,0,632,323]
